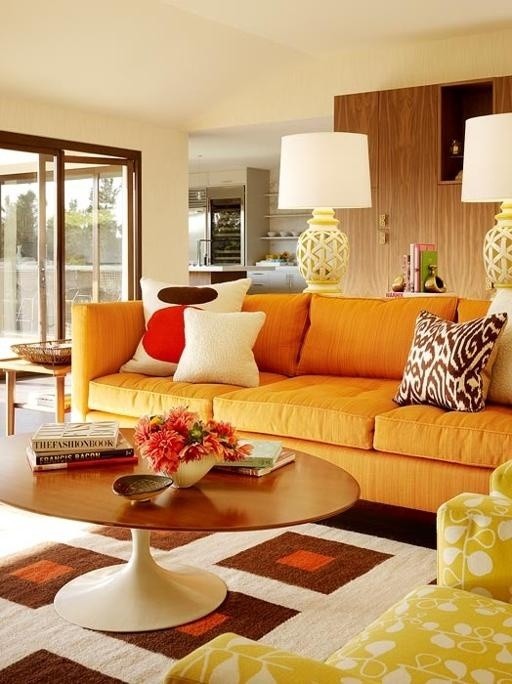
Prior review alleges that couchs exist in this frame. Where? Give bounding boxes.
[69,291,511,519]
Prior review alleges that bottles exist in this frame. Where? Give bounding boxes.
[450,139,459,154]
[256,251,296,267]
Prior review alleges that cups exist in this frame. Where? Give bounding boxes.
[265,231,301,236]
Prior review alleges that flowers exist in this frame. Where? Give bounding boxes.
[135,405,251,475]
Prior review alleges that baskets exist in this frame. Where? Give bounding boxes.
[9,337,72,366]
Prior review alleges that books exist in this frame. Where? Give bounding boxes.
[25,420,138,471]
[215,438,296,477]
[403,243,438,293]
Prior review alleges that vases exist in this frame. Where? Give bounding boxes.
[161,445,219,488]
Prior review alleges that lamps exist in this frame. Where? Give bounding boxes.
[276,132,375,294]
[458,111,512,289]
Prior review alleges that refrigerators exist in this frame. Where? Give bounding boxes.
[187,184,244,266]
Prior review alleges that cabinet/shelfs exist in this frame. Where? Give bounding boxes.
[263,191,313,260]
[247,264,275,293]
[275,266,308,293]
[332,84,440,292]
[433,71,512,289]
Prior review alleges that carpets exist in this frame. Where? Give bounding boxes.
[1,499,442,682]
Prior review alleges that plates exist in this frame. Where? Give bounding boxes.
[112,475,174,505]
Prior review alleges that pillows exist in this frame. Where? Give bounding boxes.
[170,307,266,390]
[116,275,255,378]
[483,288,512,406]
[391,308,510,413]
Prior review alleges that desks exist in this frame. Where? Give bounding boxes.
[1,358,72,435]
[1,417,363,634]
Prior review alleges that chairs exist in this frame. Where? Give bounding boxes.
[485,456,512,498]
[165,489,511,683]
